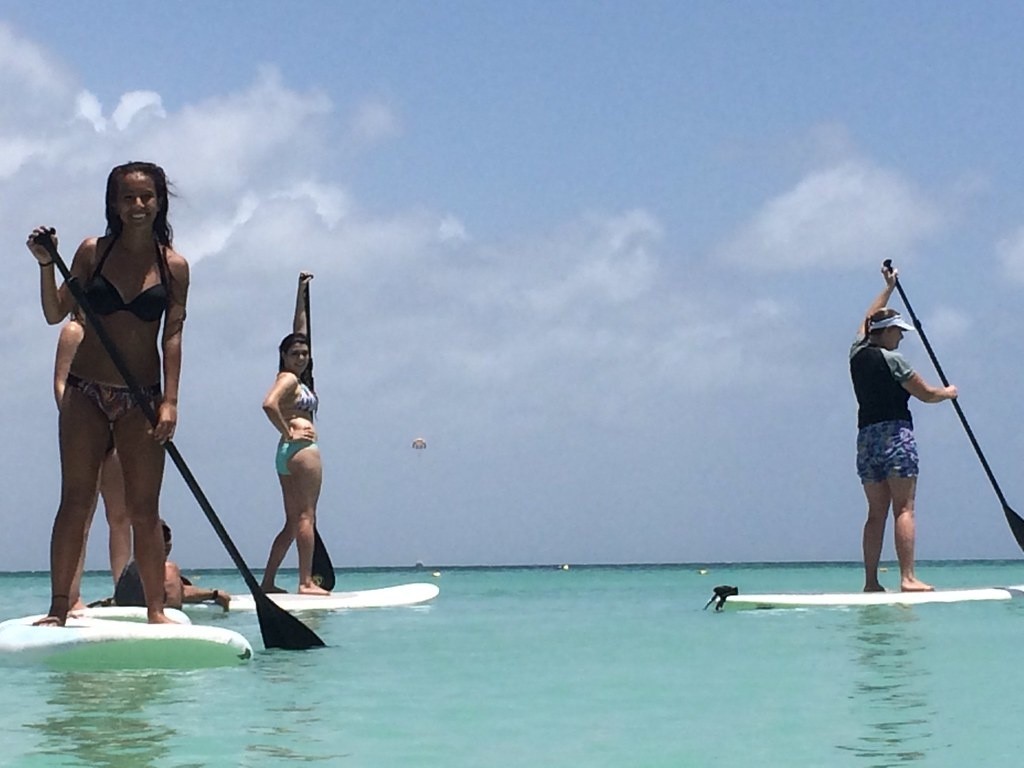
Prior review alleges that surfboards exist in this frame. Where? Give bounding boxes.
[1,605,255,668]
[712,583,1023,608]
[93,581,440,615]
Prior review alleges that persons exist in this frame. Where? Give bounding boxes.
[25,162,231,628]
[260,271,332,595]
[849,265,958,590]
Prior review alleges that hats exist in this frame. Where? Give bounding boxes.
[868,315,916,331]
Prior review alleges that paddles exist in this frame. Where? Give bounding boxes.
[881,257,1024,554]
[299,273,336,593]
[32,224,327,651]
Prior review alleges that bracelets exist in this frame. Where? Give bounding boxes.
[211,587,218,600]
[38,262,55,267]
